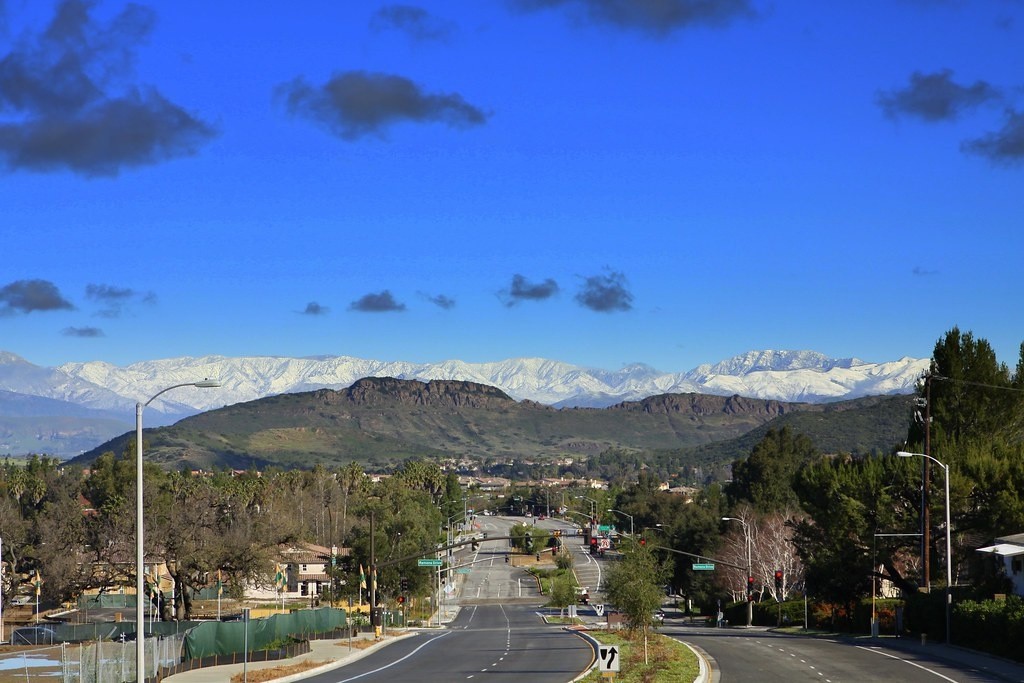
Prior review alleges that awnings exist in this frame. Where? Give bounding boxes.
[974,544,1024,558]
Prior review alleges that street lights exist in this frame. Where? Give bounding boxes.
[561,496,597,537]
[608,509,633,553]
[136,378,221,683]
[447,510,473,613]
[371,492,442,633]
[722,517,752,625]
[465,496,483,538]
[898,452,950,645]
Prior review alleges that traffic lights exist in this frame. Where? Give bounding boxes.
[552,544,556,556]
[399,597,404,604]
[775,570,783,588]
[590,538,597,555]
[748,577,754,591]
[641,537,646,549]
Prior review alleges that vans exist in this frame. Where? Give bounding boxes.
[9,627,63,645]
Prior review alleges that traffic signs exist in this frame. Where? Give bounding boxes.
[418,559,443,566]
[456,569,471,573]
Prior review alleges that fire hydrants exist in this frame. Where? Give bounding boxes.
[921,633,927,645]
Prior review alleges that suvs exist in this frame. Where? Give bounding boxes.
[525,512,531,518]
[573,587,590,605]
[651,610,664,625]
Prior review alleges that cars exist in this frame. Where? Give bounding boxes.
[597,545,602,555]
[538,515,545,520]
